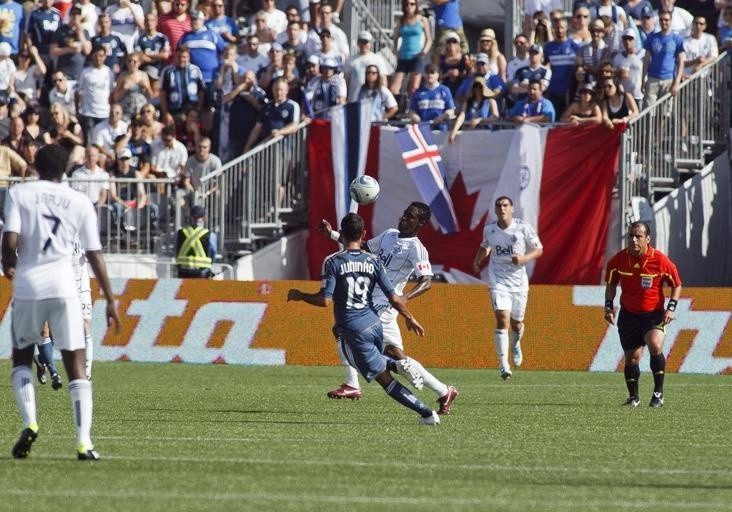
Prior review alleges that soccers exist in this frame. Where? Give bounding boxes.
[349,174,381,205]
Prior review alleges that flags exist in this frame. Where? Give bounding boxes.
[325,102,370,251]
[309,113,628,283]
[392,123,464,235]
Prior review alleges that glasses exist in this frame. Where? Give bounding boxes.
[366,70,378,75]
[49,111,59,115]
[176,3,185,6]
[20,54,30,58]
[446,40,458,44]
[600,69,612,74]
[52,78,63,83]
[592,30,602,33]
[141,109,152,113]
[198,145,210,149]
[574,14,589,19]
[270,50,284,53]
[575,71,587,76]
[514,41,528,45]
[128,59,140,64]
[623,37,633,41]
[693,21,706,26]
[288,13,299,18]
[162,135,174,141]
[474,85,483,88]
[255,19,266,23]
[426,71,436,74]
[580,91,595,95]
[603,84,617,88]
[659,18,671,21]
[212,4,222,8]
[529,51,540,54]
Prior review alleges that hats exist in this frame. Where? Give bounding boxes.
[578,83,596,93]
[588,19,606,33]
[131,113,145,125]
[528,44,544,54]
[322,57,340,68]
[0,92,11,103]
[425,63,439,72]
[476,53,490,65]
[117,148,132,159]
[479,28,496,40]
[357,30,373,42]
[641,6,654,17]
[305,54,322,65]
[446,31,461,43]
[621,27,637,40]
[189,205,206,217]
[320,28,331,38]
[25,106,39,115]
[70,3,83,14]
[188,9,205,20]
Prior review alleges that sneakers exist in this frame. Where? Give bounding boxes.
[77,447,101,461]
[436,386,459,415]
[623,396,640,406]
[327,382,363,401]
[395,359,424,392]
[649,392,664,407]
[52,375,62,389]
[422,410,441,425]
[10,423,39,459]
[34,355,48,384]
[499,367,512,380]
[510,335,523,367]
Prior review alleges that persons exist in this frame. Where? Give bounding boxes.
[316,202,459,416]
[177,11,228,153]
[242,77,301,156]
[477,27,507,76]
[0,117,34,155]
[0,41,16,103]
[388,0,434,97]
[251,0,290,34]
[305,58,346,108]
[622,0,655,32]
[255,18,276,42]
[434,0,470,71]
[87,106,131,154]
[0,186,64,390]
[48,7,93,74]
[567,6,592,52]
[358,63,399,122]
[614,29,650,112]
[47,68,79,117]
[101,0,145,52]
[597,63,627,94]
[309,1,344,21]
[73,242,93,381]
[99,151,110,170]
[677,16,721,72]
[582,18,612,70]
[604,222,682,407]
[6,96,27,119]
[543,19,582,114]
[507,81,557,124]
[306,6,349,52]
[449,77,500,144]
[284,22,310,53]
[277,52,304,87]
[511,43,552,90]
[596,0,627,28]
[134,103,164,147]
[715,0,732,50]
[258,45,285,86]
[175,207,218,278]
[134,11,171,63]
[213,49,254,107]
[72,147,112,217]
[563,81,603,126]
[110,148,146,210]
[26,0,69,49]
[288,213,441,427]
[21,139,39,165]
[112,54,153,114]
[602,78,640,128]
[305,27,345,73]
[474,198,544,379]
[178,110,211,141]
[158,0,190,52]
[3,141,123,462]
[148,124,188,180]
[654,0,695,29]
[406,64,456,126]
[0,141,28,279]
[74,0,103,37]
[182,139,224,198]
[159,47,207,122]
[440,31,472,83]
[205,0,240,46]
[91,13,127,67]
[77,46,115,126]
[9,46,47,103]
[466,54,508,99]
[44,101,84,145]
[115,118,154,172]
[345,29,391,100]
[49,1,73,18]
[508,34,530,81]
[22,108,42,139]
[636,11,685,108]
[530,9,554,45]
[0,0,23,67]
[282,5,302,23]
